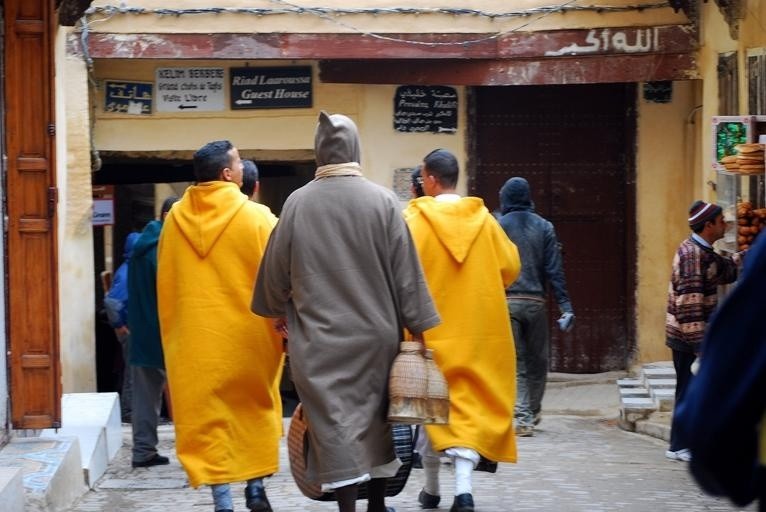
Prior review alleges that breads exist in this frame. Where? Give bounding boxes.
[717,144,765,175]
[722,202,766,252]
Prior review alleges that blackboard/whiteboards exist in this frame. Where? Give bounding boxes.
[392,85,457,134]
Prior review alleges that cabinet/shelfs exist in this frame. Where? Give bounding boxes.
[716,170,765,257]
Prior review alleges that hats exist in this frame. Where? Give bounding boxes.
[498,176,535,213]
[686,198,722,232]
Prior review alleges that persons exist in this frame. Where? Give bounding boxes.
[105,231,142,422]
[239,159,259,200]
[666,200,750,463]
[672,226,766,512]
[488,176,574,437]
[397,150,521,512]
[124,198,178,467]
[157,140,287,512]
[249,109,440,512]
[410,164,427,199]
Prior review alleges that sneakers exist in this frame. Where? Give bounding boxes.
[130,453,170,470]
[244,484,275,512]
[665,445,692,464]
[449,492,476,511]
[418,486,442,508]
